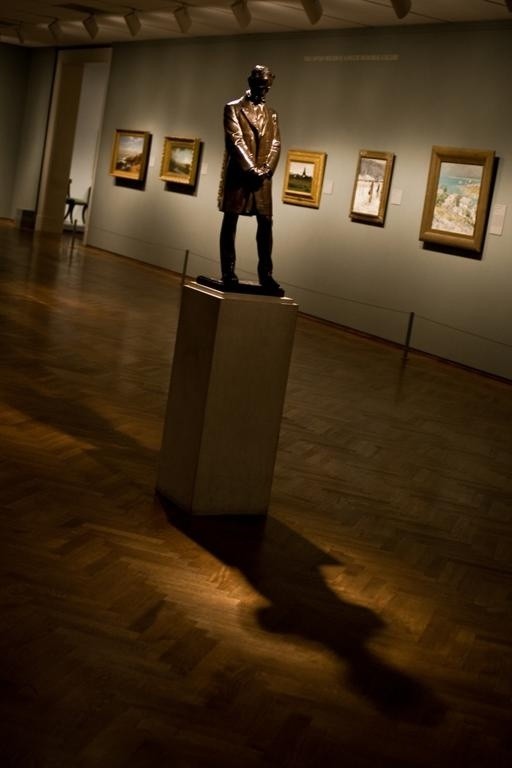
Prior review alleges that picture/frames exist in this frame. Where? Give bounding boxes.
[157,133,202,194]
[416,143,497,257]
[108,125,152,186]
[347,149,396,228]
[280,148,327,210]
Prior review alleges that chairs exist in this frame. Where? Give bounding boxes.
[62,178,88,227]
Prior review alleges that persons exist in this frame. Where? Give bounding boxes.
[218,64,281,288]
